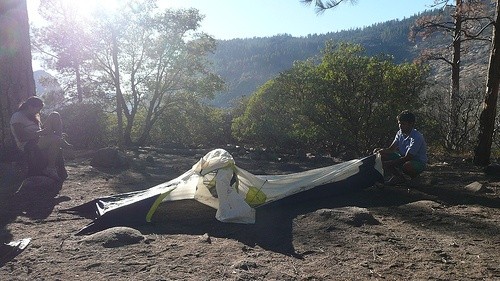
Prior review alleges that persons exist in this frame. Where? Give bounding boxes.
[373,110,427,185]
[10,97,73,178]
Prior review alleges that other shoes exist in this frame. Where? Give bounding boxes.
[385,173,408,187]
